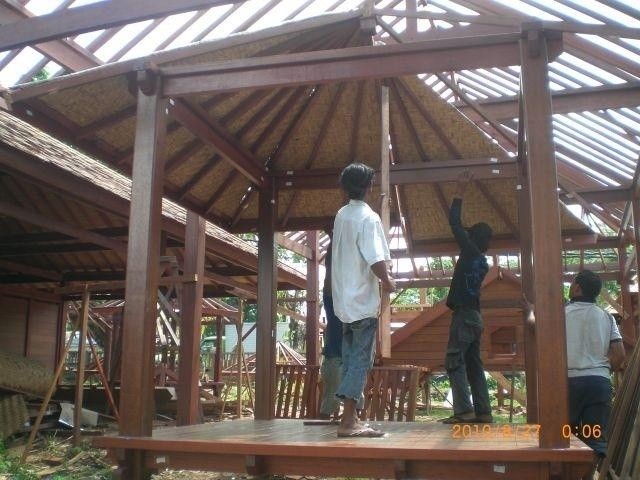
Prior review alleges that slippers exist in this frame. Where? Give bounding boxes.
[477,412,492,423]
[336,421,386,438]
[442,414,478,424]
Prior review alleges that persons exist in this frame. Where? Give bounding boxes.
[516,266,625,456]
[434,165,495,425]
[332,161,399,437]
[317,226,345,418]
